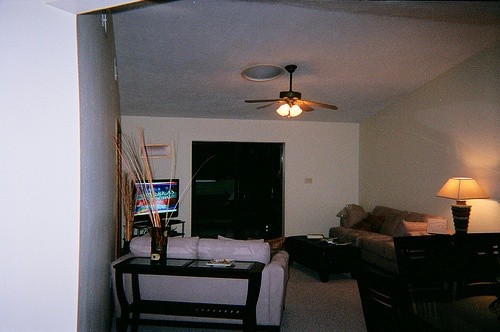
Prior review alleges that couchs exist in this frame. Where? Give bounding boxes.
[110,236,289,331]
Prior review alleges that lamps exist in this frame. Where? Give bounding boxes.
[242,65,339,118]
[435,177,488,234]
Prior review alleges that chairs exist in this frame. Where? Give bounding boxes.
[394,236,452,299]
[453,233,500,313]
[356,268,443,332]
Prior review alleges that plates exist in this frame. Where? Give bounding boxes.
[206,258,236,267]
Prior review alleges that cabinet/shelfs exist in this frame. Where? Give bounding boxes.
[132,218,185,239]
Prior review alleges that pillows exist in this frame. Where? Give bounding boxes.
[393,218,427,236]
[352,215,385,232]
[328,205,447,272]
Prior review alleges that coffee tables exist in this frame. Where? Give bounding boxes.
[283,234,364,280]
[111,259,266,332]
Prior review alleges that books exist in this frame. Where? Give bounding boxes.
[306,233,324,239]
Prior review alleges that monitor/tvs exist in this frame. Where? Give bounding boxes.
[132,179,179,222]
